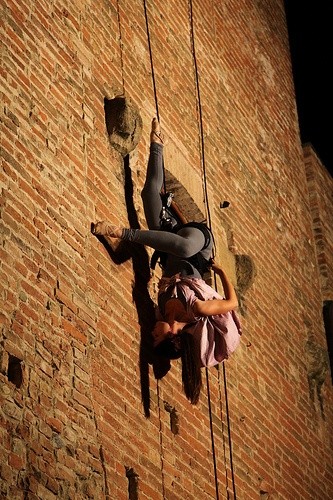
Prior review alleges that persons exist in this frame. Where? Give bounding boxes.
[92,117,244,405]
